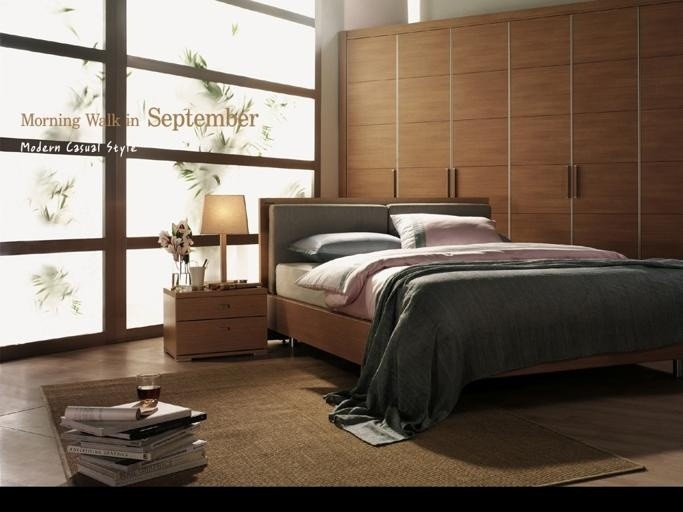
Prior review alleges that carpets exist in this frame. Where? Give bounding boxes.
[40,356,646,487]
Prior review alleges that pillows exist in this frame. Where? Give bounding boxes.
[287,231,403,264]
[391,212,503,249]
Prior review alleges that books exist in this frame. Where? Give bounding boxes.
[209,282,263,290]
[59,399,207,488]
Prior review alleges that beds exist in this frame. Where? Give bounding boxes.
[259,196,683,418]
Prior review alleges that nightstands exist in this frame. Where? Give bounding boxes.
[162,287,269,362]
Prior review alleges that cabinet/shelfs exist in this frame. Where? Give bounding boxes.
[346,35,397,199]
[640,2,682,260]
[508,7,639,258]
[397,21,510,245]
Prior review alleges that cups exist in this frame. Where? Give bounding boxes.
[136,373,159,407]
[187,267,207,286]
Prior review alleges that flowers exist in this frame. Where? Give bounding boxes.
[157,219,194,282]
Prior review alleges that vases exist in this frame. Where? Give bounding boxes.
[174,261,192,286]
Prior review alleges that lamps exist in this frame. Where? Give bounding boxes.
[199,194,251,285]
